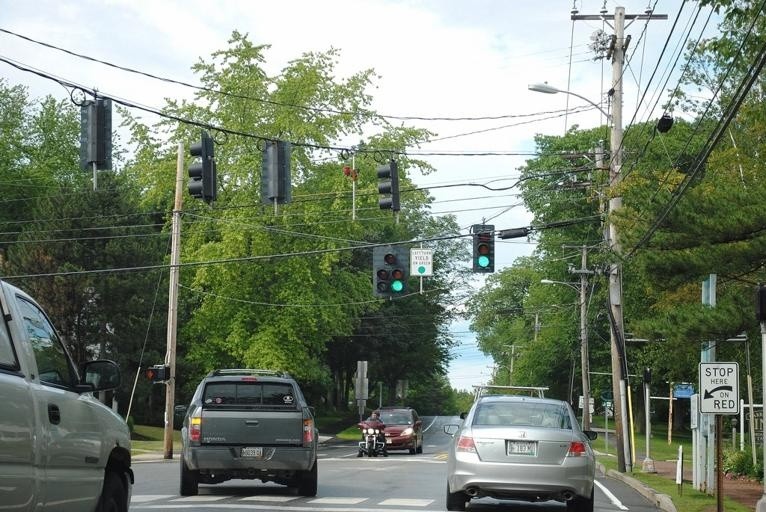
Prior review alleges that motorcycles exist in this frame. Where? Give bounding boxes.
[354,423,388,459]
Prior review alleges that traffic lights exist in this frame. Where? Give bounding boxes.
[185,128,218,203]
[473,224,494,272]
[373,247,408,297]
[147,368,157,380]
[164,367,170,379]
[374,158,397,212]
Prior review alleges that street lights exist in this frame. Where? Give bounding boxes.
[541,278,592,432]
[527,80,632,473]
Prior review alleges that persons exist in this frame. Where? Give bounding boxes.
[355,411,389,457]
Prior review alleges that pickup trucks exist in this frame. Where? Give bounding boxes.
[175,366,319,498]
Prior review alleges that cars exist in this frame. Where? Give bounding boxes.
[441,391,599,511]
[356,406,425,452]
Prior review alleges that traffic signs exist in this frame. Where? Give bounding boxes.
[410,248,433,276]
[700,363,740,414]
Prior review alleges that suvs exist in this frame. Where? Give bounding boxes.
[1,277,139,511]
